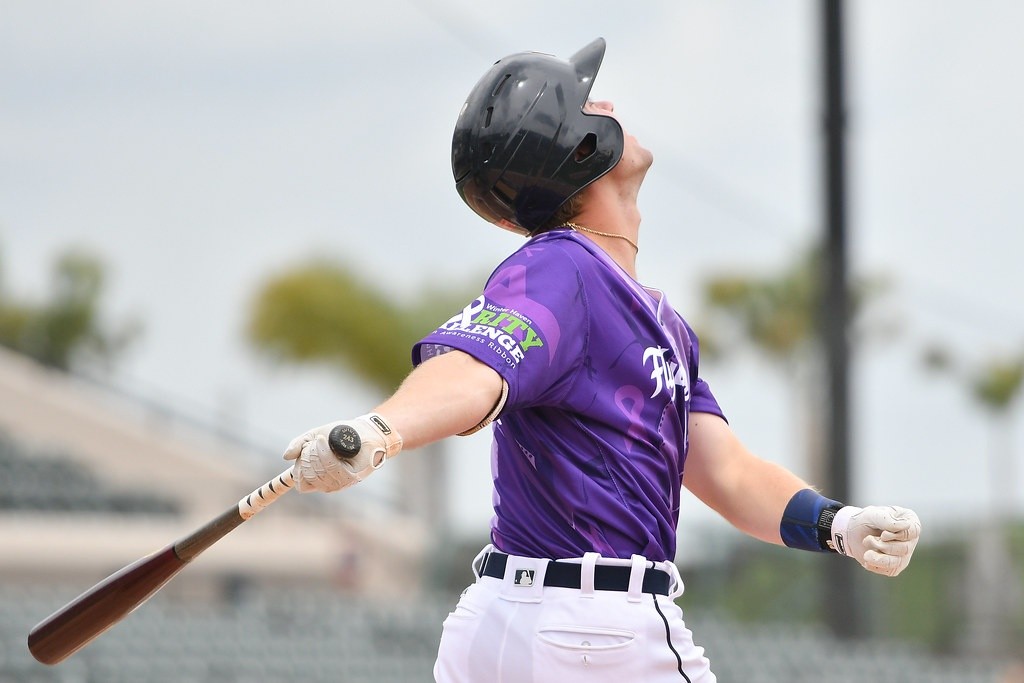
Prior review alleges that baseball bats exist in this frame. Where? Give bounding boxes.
[26,421,364,668]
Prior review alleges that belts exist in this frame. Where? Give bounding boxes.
[476,551,671,596]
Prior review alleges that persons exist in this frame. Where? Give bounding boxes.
[281,35,921,683]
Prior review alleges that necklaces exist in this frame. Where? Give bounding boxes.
[555,221,641,254]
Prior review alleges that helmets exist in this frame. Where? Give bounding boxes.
[451,37,623,237]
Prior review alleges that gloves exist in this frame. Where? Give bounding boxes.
[284,412,402,495]
[831,504,921,576]
